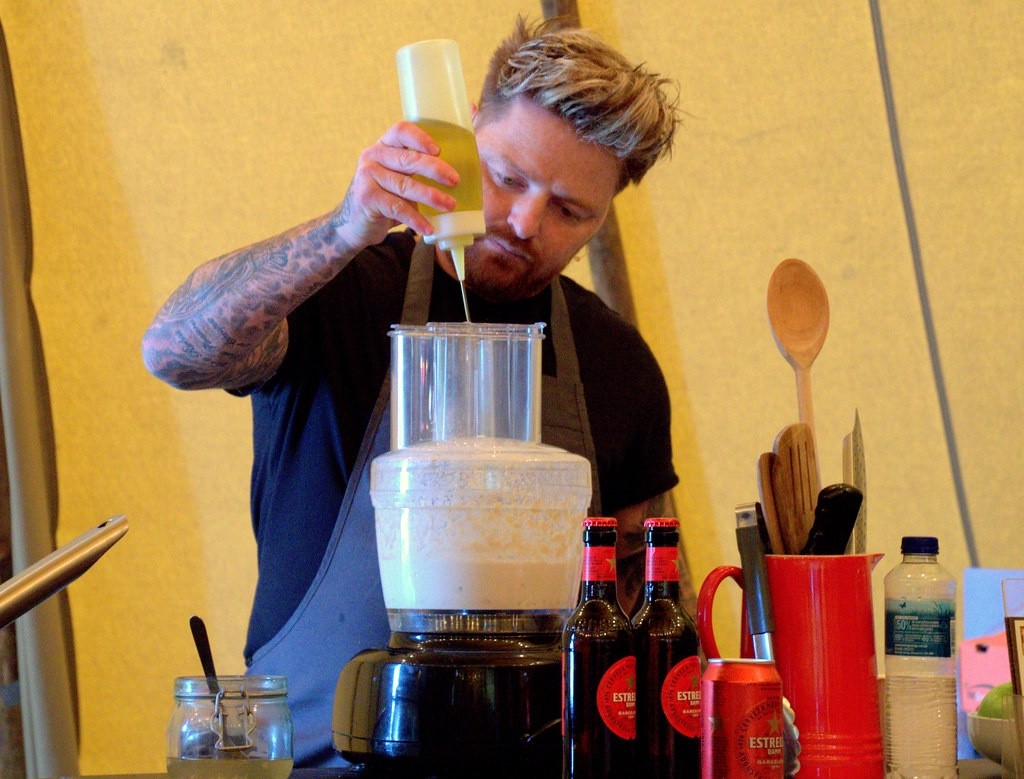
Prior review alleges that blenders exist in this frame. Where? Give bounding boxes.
[332,321,594,779]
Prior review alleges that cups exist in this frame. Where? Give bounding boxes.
[165,674,294,779]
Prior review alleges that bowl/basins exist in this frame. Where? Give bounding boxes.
[967,711,1016,774]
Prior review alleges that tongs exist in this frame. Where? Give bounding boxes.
[734,500,801,779]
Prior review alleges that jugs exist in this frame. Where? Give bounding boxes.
[695,551,886,779]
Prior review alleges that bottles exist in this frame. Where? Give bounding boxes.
[627,517,703,779]
[882,536,960,779]
[395,37,488,283]
[561,516,636,779]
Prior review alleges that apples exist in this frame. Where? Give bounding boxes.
[977,681,1020,720]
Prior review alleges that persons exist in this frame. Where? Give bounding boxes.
[140,14,680,768]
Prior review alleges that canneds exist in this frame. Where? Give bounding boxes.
[701,658,785,779]
